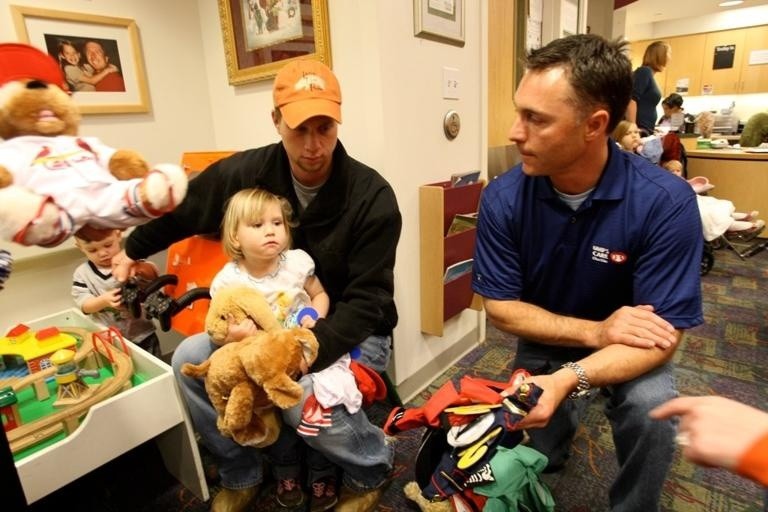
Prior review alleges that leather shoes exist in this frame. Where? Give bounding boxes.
[209,474,264,512]
[334,479,386,512]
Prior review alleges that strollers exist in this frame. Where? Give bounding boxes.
[642,136,766,280]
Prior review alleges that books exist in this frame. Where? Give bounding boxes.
[450,169,481,187]
[447,212,478,235]
[445,258,474,282]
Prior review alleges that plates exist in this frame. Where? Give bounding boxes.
[710,142,729,149]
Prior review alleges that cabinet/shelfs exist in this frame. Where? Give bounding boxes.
[700,25,768,95]
[630,32,708,98]
[420,180,484,338]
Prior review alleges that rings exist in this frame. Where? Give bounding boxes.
[675,429,691,448]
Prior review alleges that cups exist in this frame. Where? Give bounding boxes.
[683,119,694,135]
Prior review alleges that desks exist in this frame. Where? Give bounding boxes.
[0,309,211,505]
[685,145,768,240]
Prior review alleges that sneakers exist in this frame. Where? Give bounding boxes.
[275,477,304,507]
[308,477,339,512]
[744,211,759,221]
[745,220,766,234]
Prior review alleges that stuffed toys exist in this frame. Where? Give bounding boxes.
[111,261,178,332]
[0,42,190,249]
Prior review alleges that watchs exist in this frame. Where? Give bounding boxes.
[561,360,592,401]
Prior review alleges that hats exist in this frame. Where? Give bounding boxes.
[271,56,345,131]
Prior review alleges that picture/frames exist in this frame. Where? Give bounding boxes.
[413,0,466,48]
[10,5,150,115]
[215,0,333,87]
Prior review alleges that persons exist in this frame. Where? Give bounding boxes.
[57,39,117,93]
[71,229,162,361]
[83,41,125,92]
[472,32,708,512]
[662,158,683,176]
[645,392,768,488]
[110,59,404,512]
[613,119,765,244]
[625,41,672,138]
[657,93,687,134]
[209,186,329,347]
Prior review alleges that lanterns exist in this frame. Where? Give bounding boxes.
[180,284,320,450]
